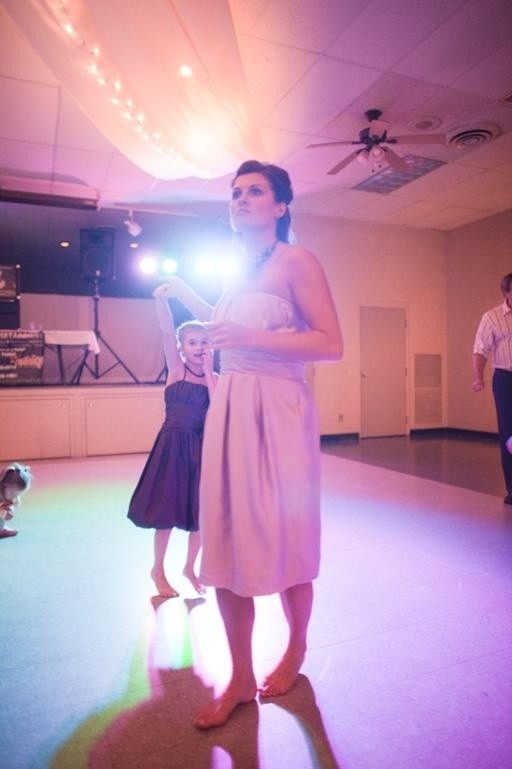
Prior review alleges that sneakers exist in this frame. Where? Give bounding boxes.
[1,527,17,537]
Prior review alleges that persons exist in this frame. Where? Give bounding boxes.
[471,270,512,507]
[163,158,345,734]
[126,283,220,601]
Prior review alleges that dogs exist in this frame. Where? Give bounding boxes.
[0,460,31,537]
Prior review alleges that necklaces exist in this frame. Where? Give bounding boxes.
[182,363,206,379]
[246,236,284,269]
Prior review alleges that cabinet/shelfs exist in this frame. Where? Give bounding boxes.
[0,384,168,462]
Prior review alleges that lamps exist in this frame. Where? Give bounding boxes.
[356,110,395,171]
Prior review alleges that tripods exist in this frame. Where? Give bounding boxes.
[68,281,141,384]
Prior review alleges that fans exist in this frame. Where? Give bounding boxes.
[306,109,447,176]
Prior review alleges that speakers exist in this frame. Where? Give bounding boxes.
[77,227,115,282]
